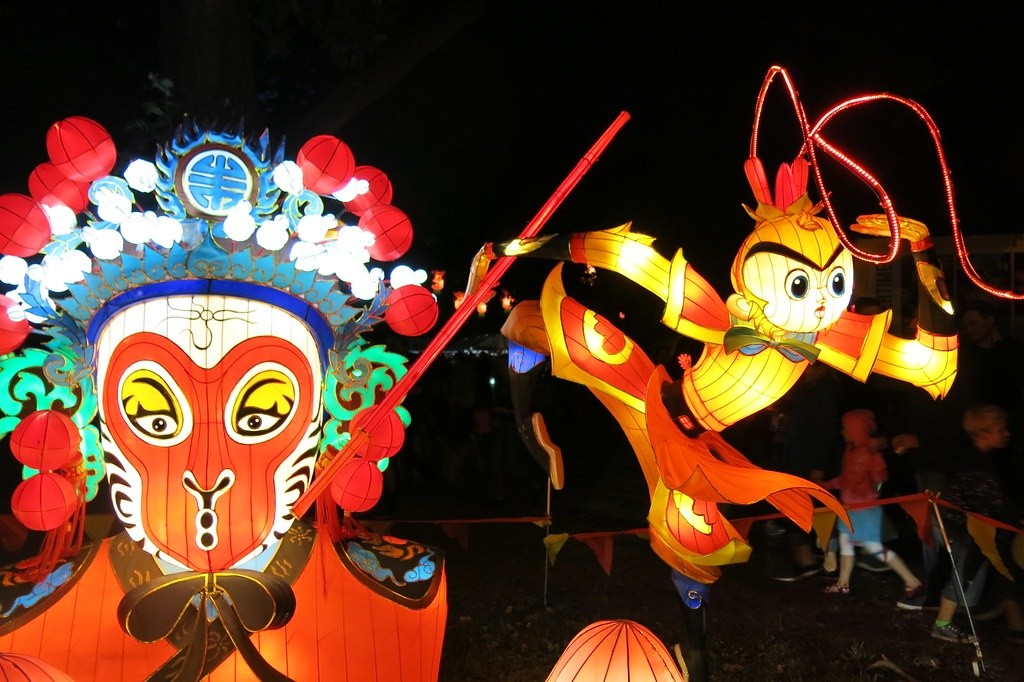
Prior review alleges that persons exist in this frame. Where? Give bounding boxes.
[768,297,1024,644]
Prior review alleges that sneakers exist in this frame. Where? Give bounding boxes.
[895,582,940,612]
[930,621,980,644]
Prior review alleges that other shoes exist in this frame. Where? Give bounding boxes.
[894,584,923,600]
[772,555,823,582]
[854,545,892,572]
[1005,627,1024,644]
[823,552,839,576]
[821,583,850,596]
[956,604,975,618]
[722,587,753,606]
